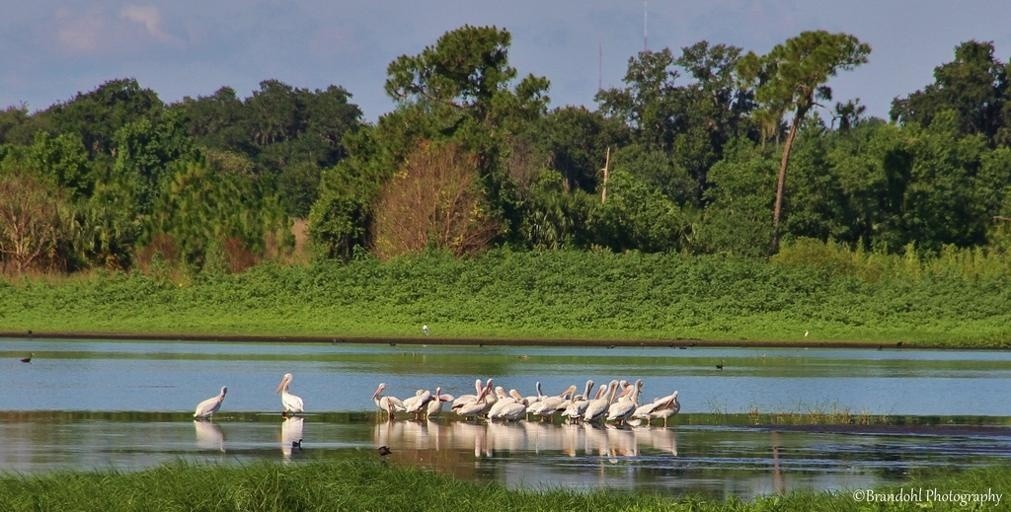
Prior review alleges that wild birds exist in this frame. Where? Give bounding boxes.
[193,386,228,418]
[292,439,303,447]
[275,373,305,413]
[370,383,407,418]
[403,377,680,430]
[378,446,392,455]
